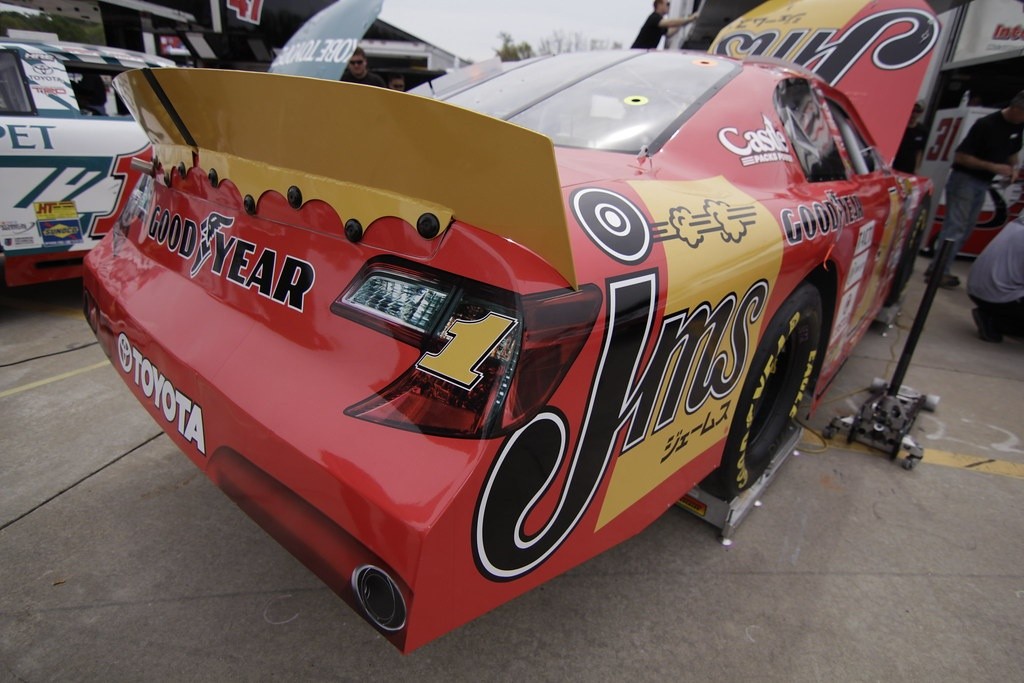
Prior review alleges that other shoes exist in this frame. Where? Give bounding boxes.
[972,307,1004,343]
[924,269,960,286]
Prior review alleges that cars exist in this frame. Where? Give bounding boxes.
[82,1,945,653]
[1,1,382,289]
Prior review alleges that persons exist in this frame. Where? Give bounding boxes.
[80,74,106,114]
[388,74,404,91]
[631,0,699,48]
[894,104,926,173]
[924,90,1024,285]
[340,47,386,88]
[967,215,1024,343]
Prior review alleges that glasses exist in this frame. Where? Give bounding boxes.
[348,60,365,66]
